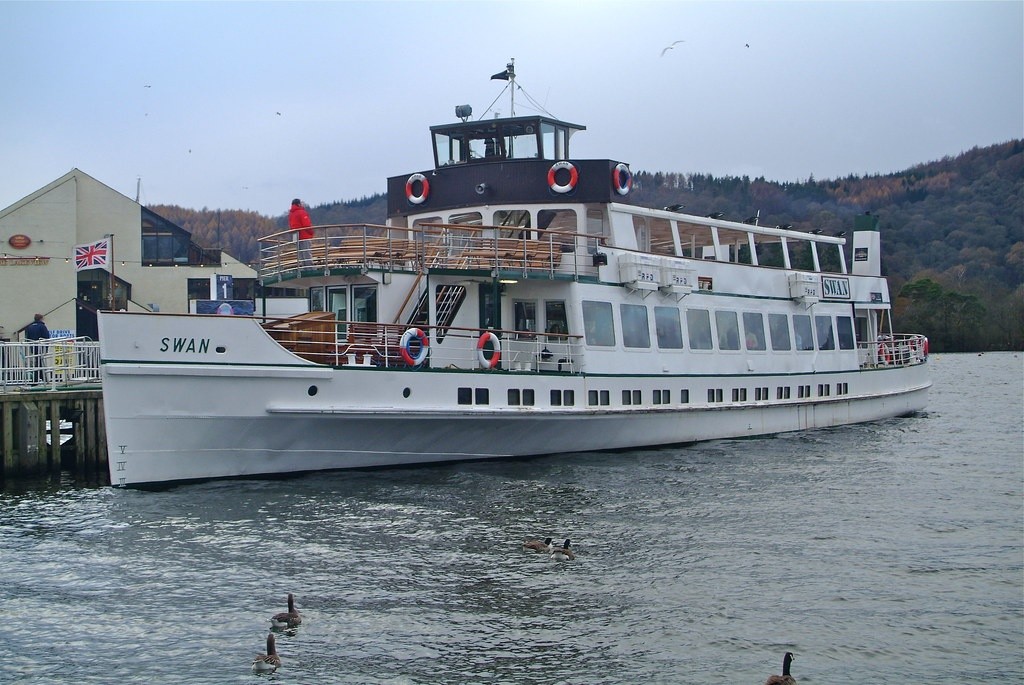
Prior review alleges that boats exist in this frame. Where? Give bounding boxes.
[95,56,930,489]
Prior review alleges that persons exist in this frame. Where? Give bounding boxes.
[25,314,49,386]
[550,324,563,340]
[288,198,314,270]
[519,329,530,338]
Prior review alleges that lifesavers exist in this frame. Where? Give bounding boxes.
[613,163,632,195]
[909,336,919,353]
[923,337,928,356]
[477,331,501,369]
[547,161,579,194]
[879,343,890,366]
[398,327,430,367]
[405,173,430,205]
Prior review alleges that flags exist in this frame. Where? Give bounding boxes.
[73,237,112,274]
